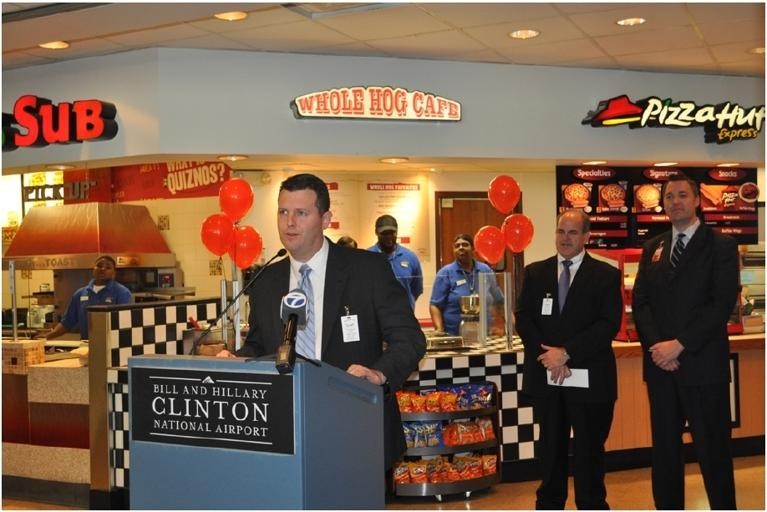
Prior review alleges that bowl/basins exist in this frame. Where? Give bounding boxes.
[738,181,761,204]
[460,295,481,315]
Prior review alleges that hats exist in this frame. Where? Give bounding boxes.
[376,215,398,234]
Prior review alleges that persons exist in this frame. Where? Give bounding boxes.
[215,173,427,473]
[367,215,423,313]
[430,233,516,337]
[32,255,133,340]
[632,175,739,510]
[337,237,357,249]
[514,209,623,510]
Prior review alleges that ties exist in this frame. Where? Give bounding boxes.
[670,233,686,269]
[292,264,317,363]
[557,260,573,314]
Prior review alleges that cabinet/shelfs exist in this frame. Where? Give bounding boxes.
[392,380,503,504]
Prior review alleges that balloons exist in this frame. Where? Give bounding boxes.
[487,175,521,213]
[219,178,254,221]
[501,213,534,254]
[228,225,262,270]
[473,225,507,266]
[201,215,238,256]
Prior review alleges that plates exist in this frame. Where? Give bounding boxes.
[561,183,663,211]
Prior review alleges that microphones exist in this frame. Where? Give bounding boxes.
[275,288,310,374]
[189,249,287,354]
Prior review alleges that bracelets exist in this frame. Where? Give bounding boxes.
[564,351,570,360]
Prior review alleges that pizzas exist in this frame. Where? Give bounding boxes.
[601,185,625,201]
[563,182,590,202]
[636,184,662,205]
